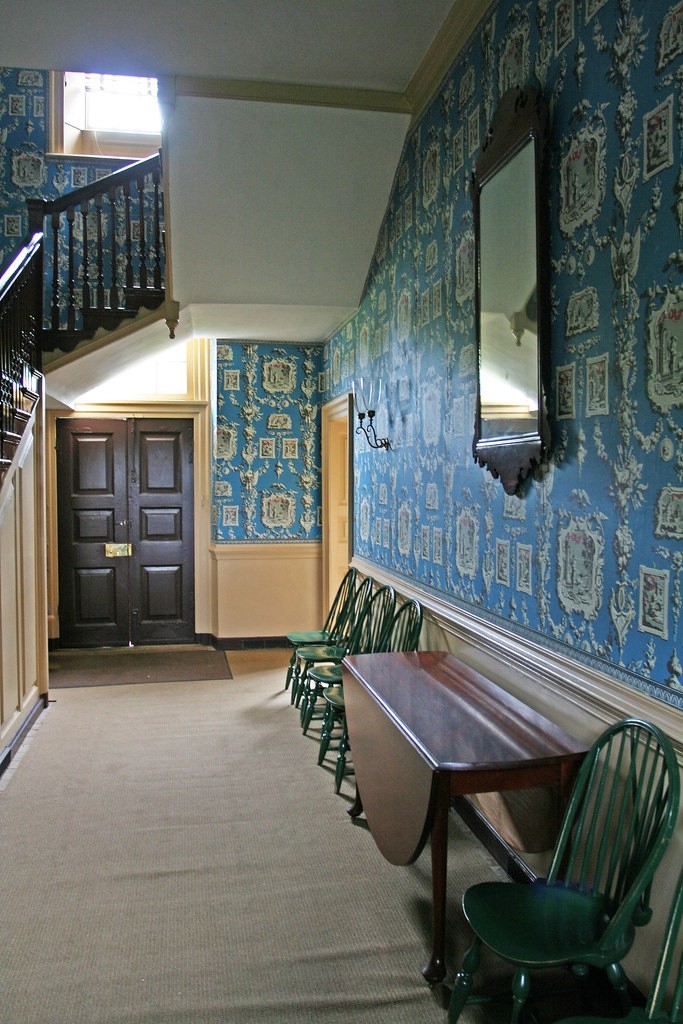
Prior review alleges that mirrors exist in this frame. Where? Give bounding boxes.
[469,85,553,497]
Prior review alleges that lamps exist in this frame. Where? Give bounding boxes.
[353,376,390,451]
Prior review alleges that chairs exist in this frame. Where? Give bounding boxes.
[295,576,374,729]
[284,567,357,705]
[445,718,683,1024]
[302,585,395,750]
[316,599,423,794]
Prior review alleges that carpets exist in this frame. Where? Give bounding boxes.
[48,650,233,689]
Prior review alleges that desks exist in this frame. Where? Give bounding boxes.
[341,652,591,990]
